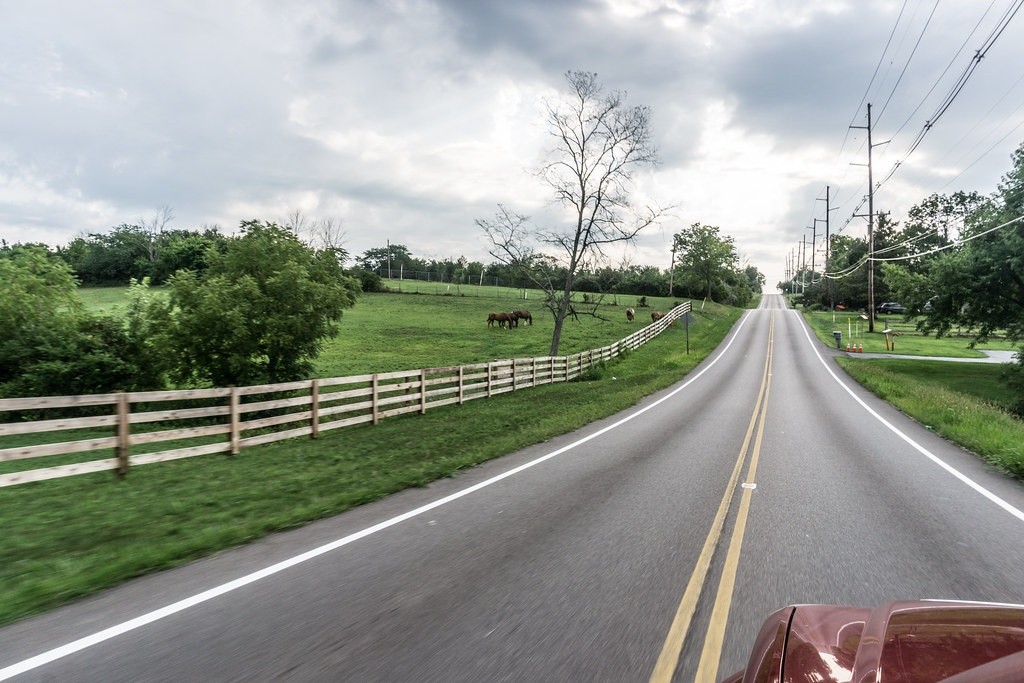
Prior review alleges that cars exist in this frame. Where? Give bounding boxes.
[719,598,1024,682]
[876,301,907,315]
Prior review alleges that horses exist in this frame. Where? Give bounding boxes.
[487,310,532,330]
[651,312,667,322]
[626,307,635,323]
